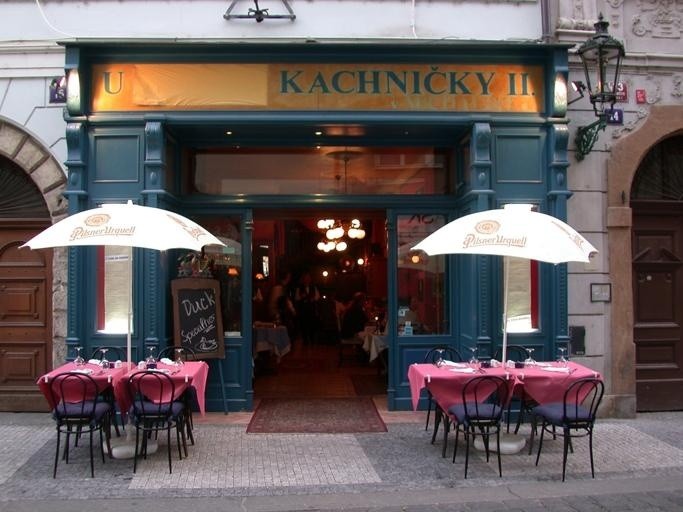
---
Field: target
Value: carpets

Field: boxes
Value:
[245,397,388,433]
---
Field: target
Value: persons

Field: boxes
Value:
[292,271,321,348]
[271,265,297,349]
[338,291,371,363]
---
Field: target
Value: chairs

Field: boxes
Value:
[50,346,198,474]
[424,344,604,483]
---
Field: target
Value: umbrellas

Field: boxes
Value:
[394,236,446,334]
[409,204,599,367]
[16,198,229,370]
[200,234,243,268]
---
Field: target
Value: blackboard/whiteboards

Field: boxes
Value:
[171,278,225,360]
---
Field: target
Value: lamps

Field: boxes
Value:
[568,10,626,161]
[53,76,65,99]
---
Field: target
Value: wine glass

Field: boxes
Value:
[524,348,537,369]
[175,348,185,369]
[144,346,157,369]
[436,349,447,369]
[469,347,480,369]
[558,347,568,368]
[73,347,86,368]
[98,349,111,370]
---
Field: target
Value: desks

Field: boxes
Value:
[410,361,513,459]
